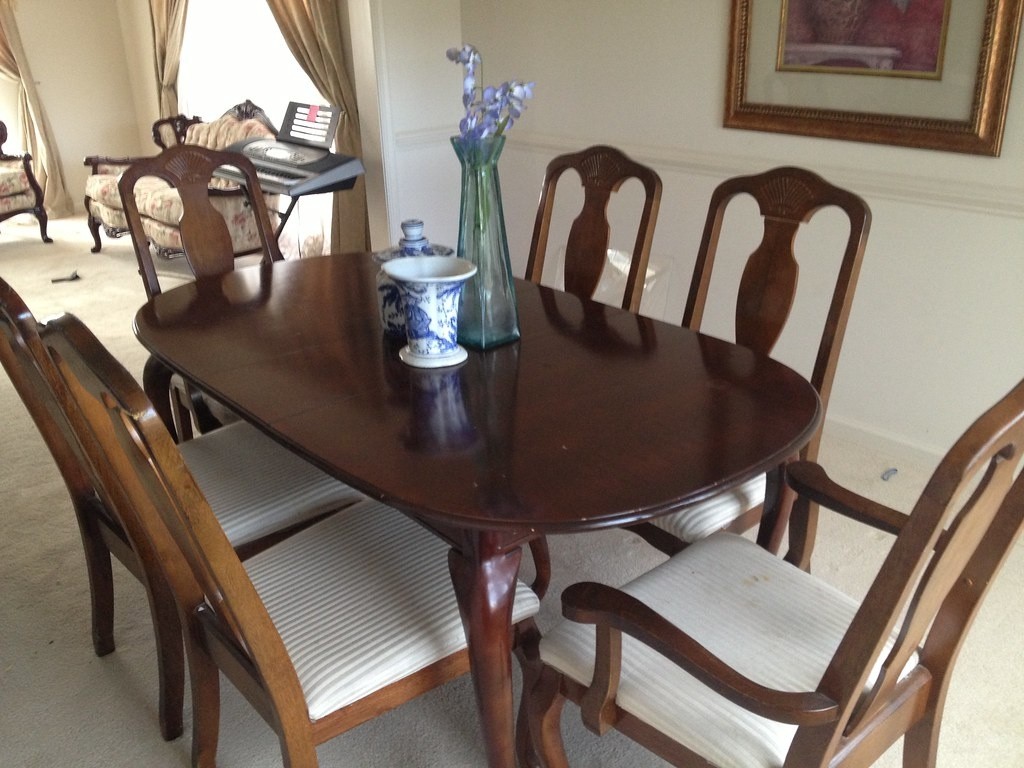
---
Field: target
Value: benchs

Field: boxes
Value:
[82,98,278,260]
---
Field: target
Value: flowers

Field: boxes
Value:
[445,42,535,140]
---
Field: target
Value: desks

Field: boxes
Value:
[132,253,823,768]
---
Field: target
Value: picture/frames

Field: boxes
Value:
[721,0,1024,158]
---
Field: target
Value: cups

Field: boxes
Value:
[383,256,477,368]
[371,220,454,337]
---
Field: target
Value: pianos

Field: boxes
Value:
[210,99,366,241]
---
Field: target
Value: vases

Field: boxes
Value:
[448,133,522,351]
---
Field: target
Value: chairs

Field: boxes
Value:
[39,310,542,768]
[527,378,1024,768]
[525,143,664,315]
[0,120,55,244]
[116,144,286,443]
[524,167,874,574]
[0,279,367,742]
